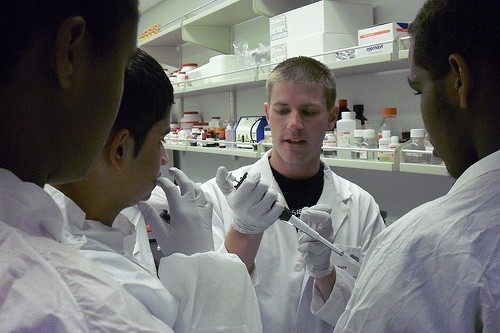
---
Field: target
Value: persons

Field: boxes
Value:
[323,0,500,333]
[198,55,386,333]
[39,47,264,333]
[0,0,179,333]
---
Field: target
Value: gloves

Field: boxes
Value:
[297,203,333,277]
[216,166,284,234]
[138,166,215,256]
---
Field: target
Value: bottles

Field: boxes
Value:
[176,68,186,88]
[336,111,361,159]
[180,111,202,130]
[376,108,402,143]
[322,128,337,158]
[169,68,178,90]
[225,123,234,148]
[352,105,368,130]
[206,130,226,148]
[351,130,365,160]
[403,128,426,163]
[360,129,378,160]
[379,130,399,162]
[334,99,352,128]
[209,116,222,127]
[181,63,199,74]
[167,122,180,144]
[163,69,170,79]
[177,122,208,146]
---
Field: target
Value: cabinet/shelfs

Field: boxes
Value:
[134,0,451,180]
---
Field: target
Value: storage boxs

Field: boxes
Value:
[357,22,411,56]
[270,33,358,73]
[268,0,376,44]
